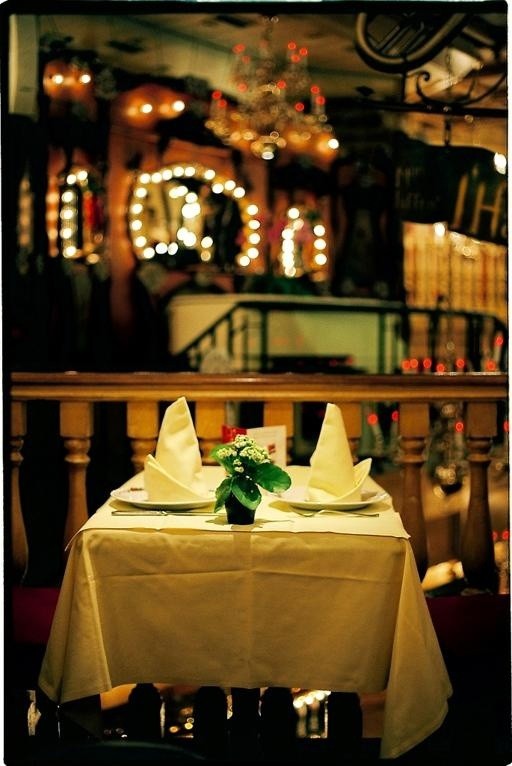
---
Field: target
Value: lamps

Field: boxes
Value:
[206,12,341,165]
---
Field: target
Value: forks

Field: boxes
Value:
[291,506,379,517]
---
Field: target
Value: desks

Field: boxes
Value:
[35,466,453,766]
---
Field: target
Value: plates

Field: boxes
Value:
[112,486,217,511]
[268,491,385,508]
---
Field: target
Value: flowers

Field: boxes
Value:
[210,434,291,511]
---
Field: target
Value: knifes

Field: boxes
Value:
[111,511,220,517]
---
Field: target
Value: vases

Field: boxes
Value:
[224,492,254,525]
